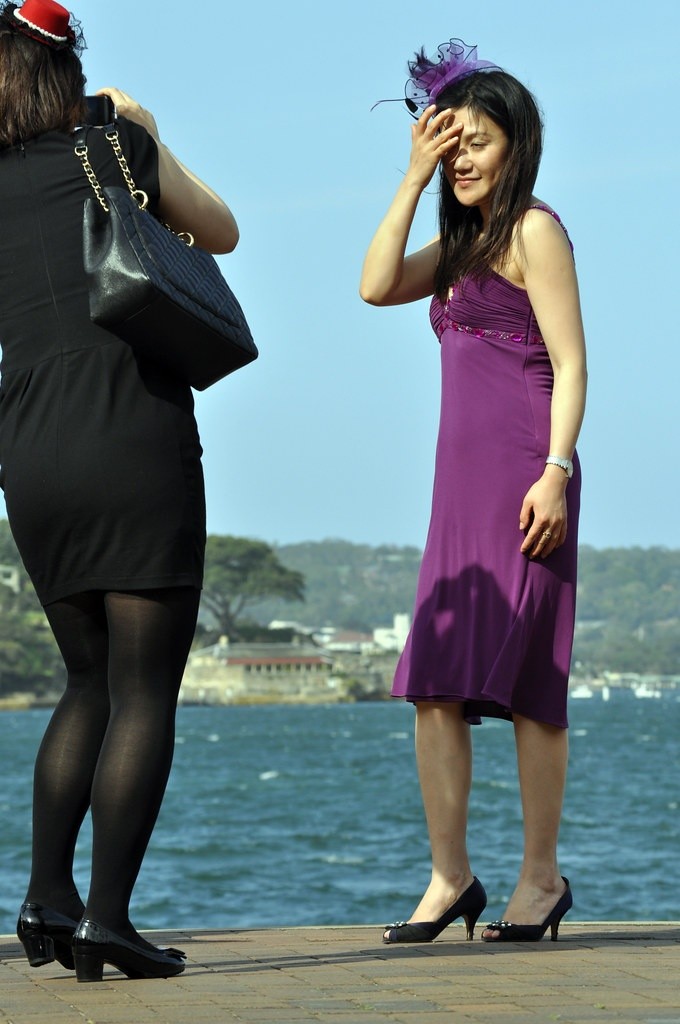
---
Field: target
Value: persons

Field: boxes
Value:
[0,0,239,977]
[361,37,586,943]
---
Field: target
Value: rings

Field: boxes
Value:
[542,532,551,539]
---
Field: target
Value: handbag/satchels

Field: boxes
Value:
[74,123,259,390]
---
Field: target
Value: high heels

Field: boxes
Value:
[71,920,188,983]
[480,876,573,943]
[15,903,85,969]
[382,876,487,943]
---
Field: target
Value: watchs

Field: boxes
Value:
[545,456,574,478]
